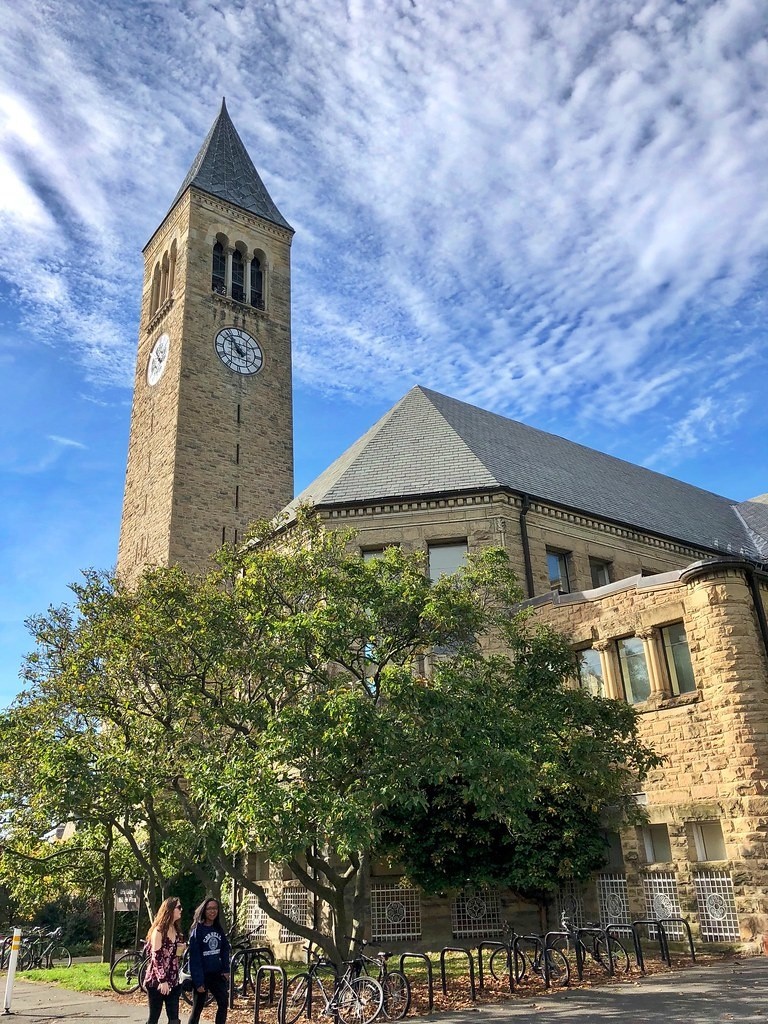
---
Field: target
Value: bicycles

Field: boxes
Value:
[342,934,411,1022]
[0,924,72,972]
[549,909,630,976]
[110,919,275,1006]
[278,946,383,1024]
[489,919,570,988]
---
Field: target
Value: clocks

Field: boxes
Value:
[145,332,170,388]
[214,326,263,375]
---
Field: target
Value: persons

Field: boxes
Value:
[144,897,186,1024]
[188,897,231,1024]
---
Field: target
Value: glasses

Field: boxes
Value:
[206,907,218,911]
[174,905,182,910]
